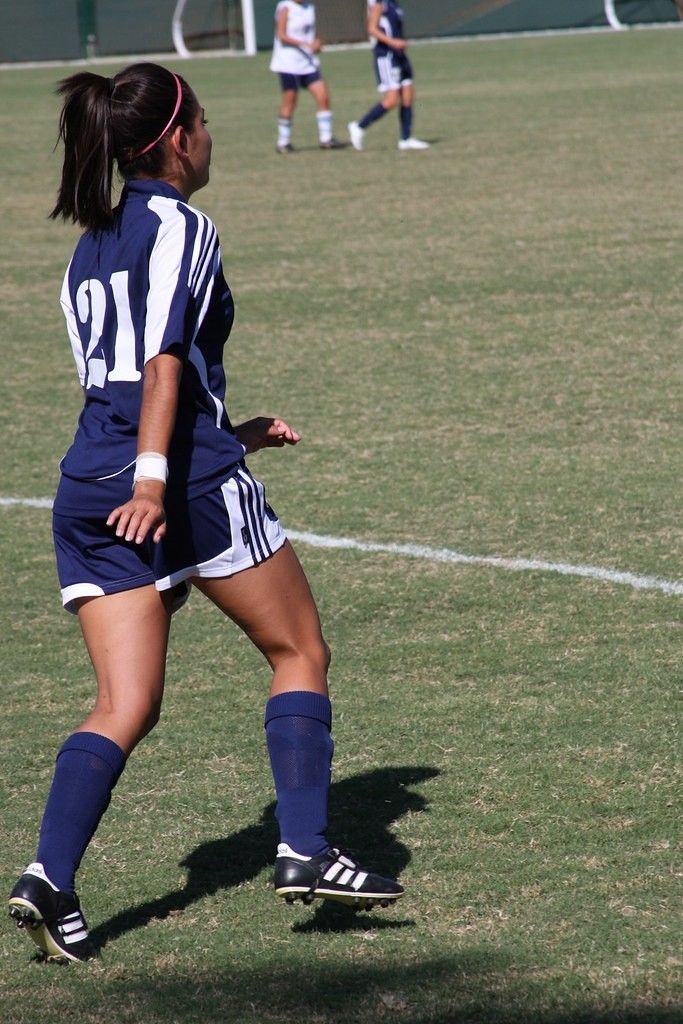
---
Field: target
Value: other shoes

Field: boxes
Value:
[319,138,348,149]
[275,144,295,154]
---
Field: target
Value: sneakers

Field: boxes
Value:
[398,137,430,151]
[347,121,365,151]
[273,842,405,911]
[8,863,101,963]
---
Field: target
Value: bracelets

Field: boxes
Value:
[132,451,169,485]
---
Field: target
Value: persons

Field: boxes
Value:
[269,1,348,155]
[5,62,406,964]
[347,0,431,152]
